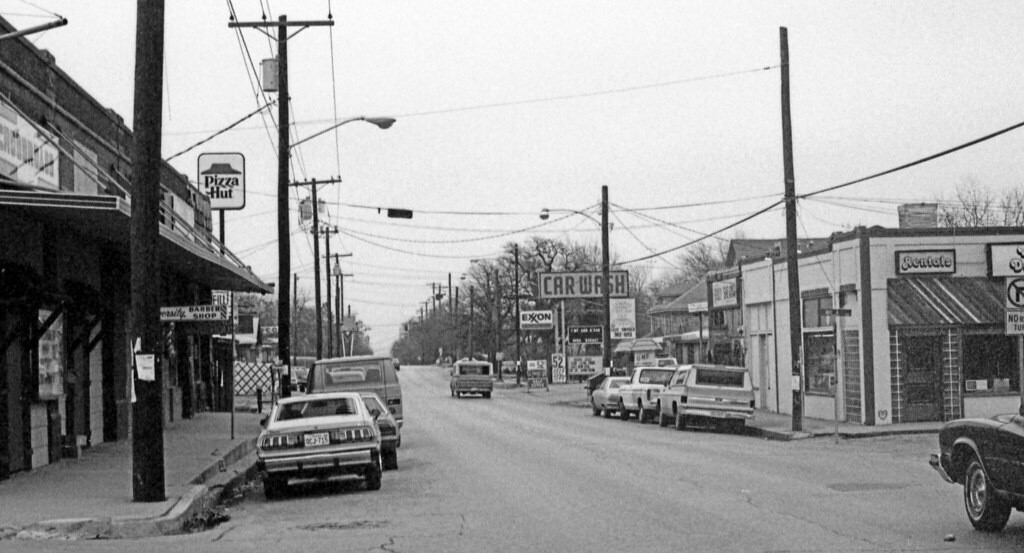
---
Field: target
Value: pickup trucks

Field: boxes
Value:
[618,366,678,422]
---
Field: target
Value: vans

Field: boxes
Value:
[656,360,755,433]
[306,355,404,448]
[450,363,494,399]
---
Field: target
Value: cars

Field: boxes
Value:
[257,392,384,499]
[814,373,835,392]
[301,392,401,470]
[392,359,400,370]
[928,408,1024,532]
[283,365,368,392]
[589,375,630,417]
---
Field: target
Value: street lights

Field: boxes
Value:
[277,114,395,398]
[540,206,611,375]
[470,258,521,386]
